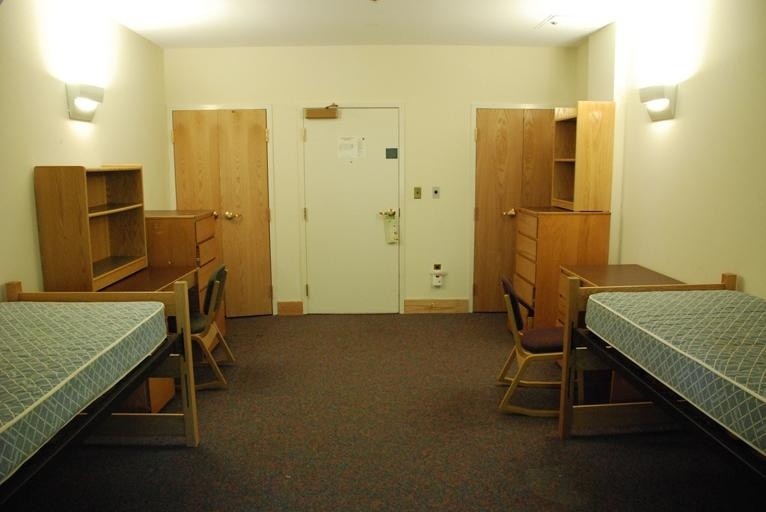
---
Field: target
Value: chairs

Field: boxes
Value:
[498,275,590,417]
[167,265,236,391]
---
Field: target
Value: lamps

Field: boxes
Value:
[67,85,103,122]
[640,86,677,123]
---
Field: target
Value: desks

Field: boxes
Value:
[557,264,689,403]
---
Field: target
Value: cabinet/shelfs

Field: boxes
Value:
[551,100,615,212]
[505,206,610,335]
[82,210,226,413]
[34,164,148,293]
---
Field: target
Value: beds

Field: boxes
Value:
[0,282,198,512]
[558,272,766,476]
